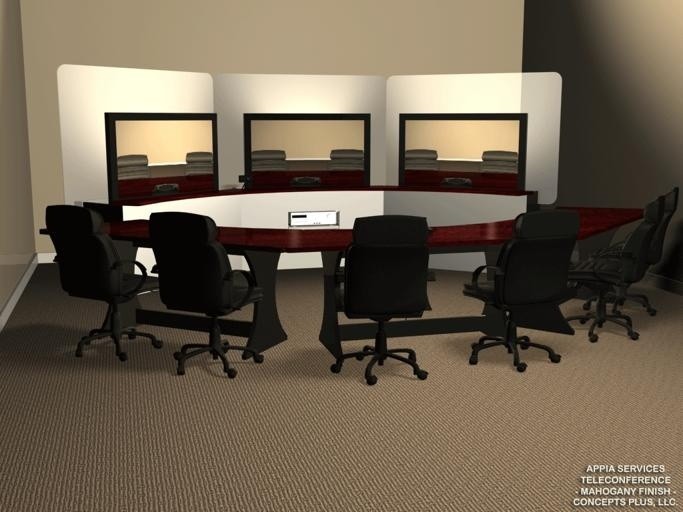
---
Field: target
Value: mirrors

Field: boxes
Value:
[104,109,219,207]
[396,111,529,187]
[241,111,371,189]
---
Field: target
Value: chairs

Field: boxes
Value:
[461,185,680,372]
[331,215,437,386]
[44,204,263,378]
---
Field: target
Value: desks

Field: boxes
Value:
[39,206,645,361]
